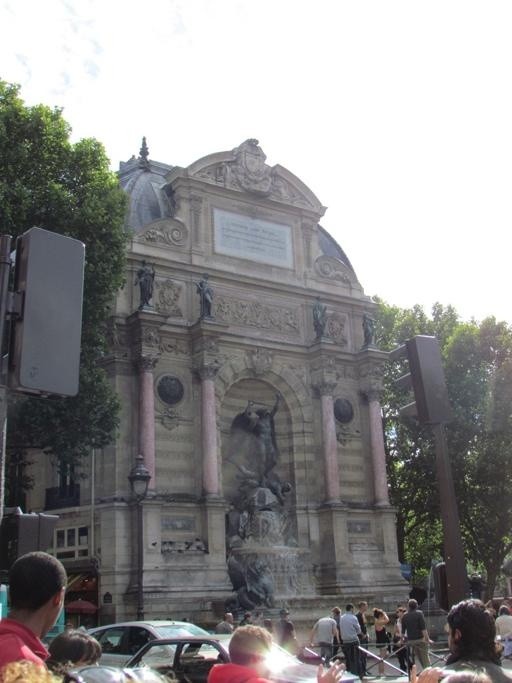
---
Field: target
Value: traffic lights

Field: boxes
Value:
[388,341,419,422]
[3,512,61,582]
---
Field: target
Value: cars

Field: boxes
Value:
[62,633,360,682]
[85,620,212,666]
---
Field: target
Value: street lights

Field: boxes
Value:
[427,557,439,638]
[125,452,153,619]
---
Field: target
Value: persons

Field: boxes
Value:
[243,390,282,485]
[361,311,377,345]
[209,624,346,682]
[485,599,511,658]
[216,612,233,633]
[438,598,511,682]
[307,598,433,683]
[311,300,328,339]
[48,630,102,672]
[1,552,67,681]
[195,277,215,317]
[223,455,293,502]
[239,610,296,646]
[132,259,158,306]
